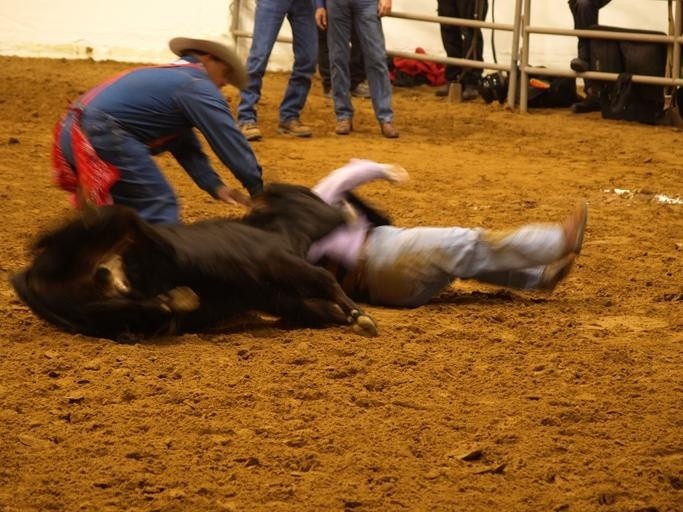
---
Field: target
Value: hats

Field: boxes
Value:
[170,31,248,91]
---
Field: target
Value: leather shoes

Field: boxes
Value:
[571,96,599,114]
[435,83,450,97]
[570,58,589,71]
[461,85,478,100]
[540,258,573,291]
[563,200,588,254]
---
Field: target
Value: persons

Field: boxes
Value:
[566,0,612,114]
[311,0,400,139]
[315,24,373,99]
[304,156,590,309]
[50,28,265,221]
[236,0,318,141]
[435,1,488,100]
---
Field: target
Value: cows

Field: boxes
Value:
[9,177,391,347]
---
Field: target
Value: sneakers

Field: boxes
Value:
[278,120,311,136]
[239,122,261,140]
[335,119,351,135]
[379,122,398,137]
